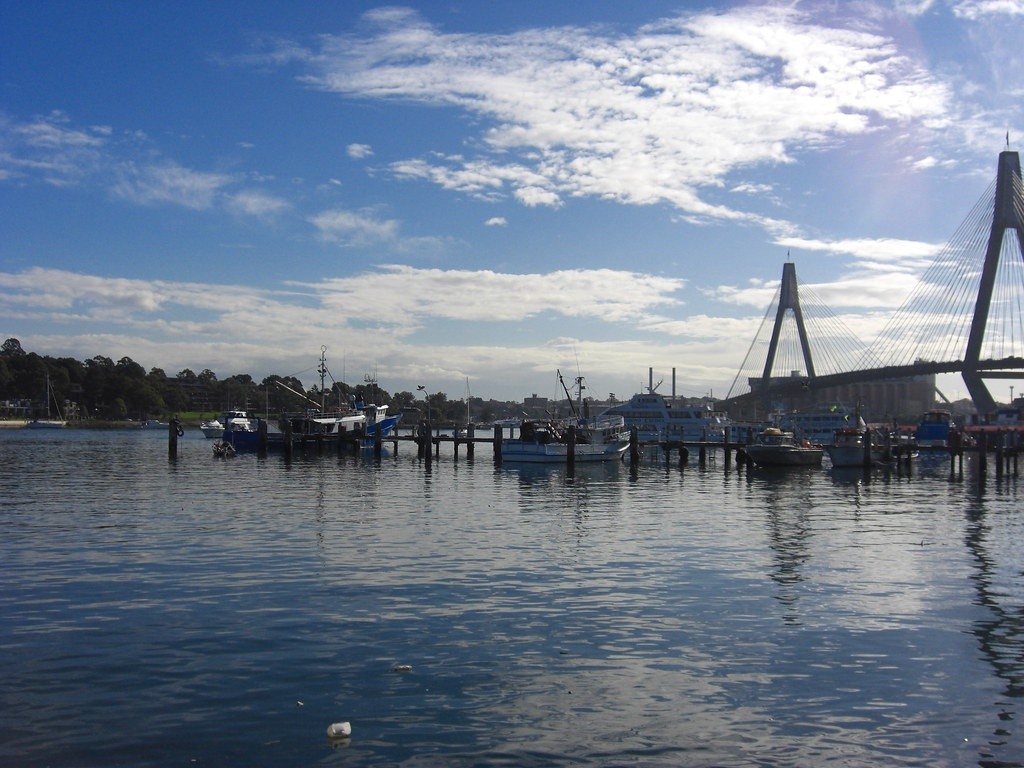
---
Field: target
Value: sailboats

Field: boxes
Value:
[27,375,72,428]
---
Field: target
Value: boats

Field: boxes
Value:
[142,419,170,429]
[887,428,921,461]
[200,402,257,439]
[500,369,632,462]
[915,410,977,451]
[223,345,406,449]
[595,380,734,443]
[730,402,888,468]
